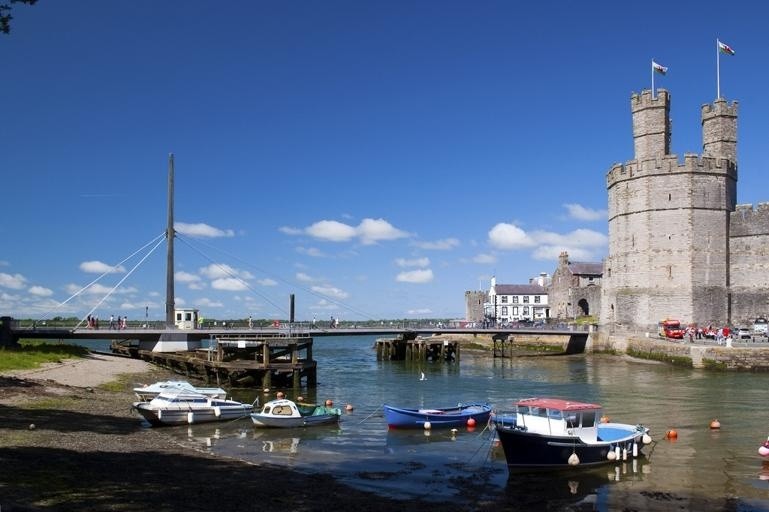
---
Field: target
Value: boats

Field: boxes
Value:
[382,397,650,468]
[132,380,342,428]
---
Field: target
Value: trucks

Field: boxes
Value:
[658,319,684,339]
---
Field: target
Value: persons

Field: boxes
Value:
[84,312,130,331]
[198,316,205,328]
[245,315,255,329]
[684,322,730,345]
[329,316,337,329]
[311,316,319,328]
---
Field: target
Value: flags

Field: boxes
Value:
[719,41,739,57]
[650,61,669,76]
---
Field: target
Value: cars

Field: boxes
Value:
[733,327,751,339]
